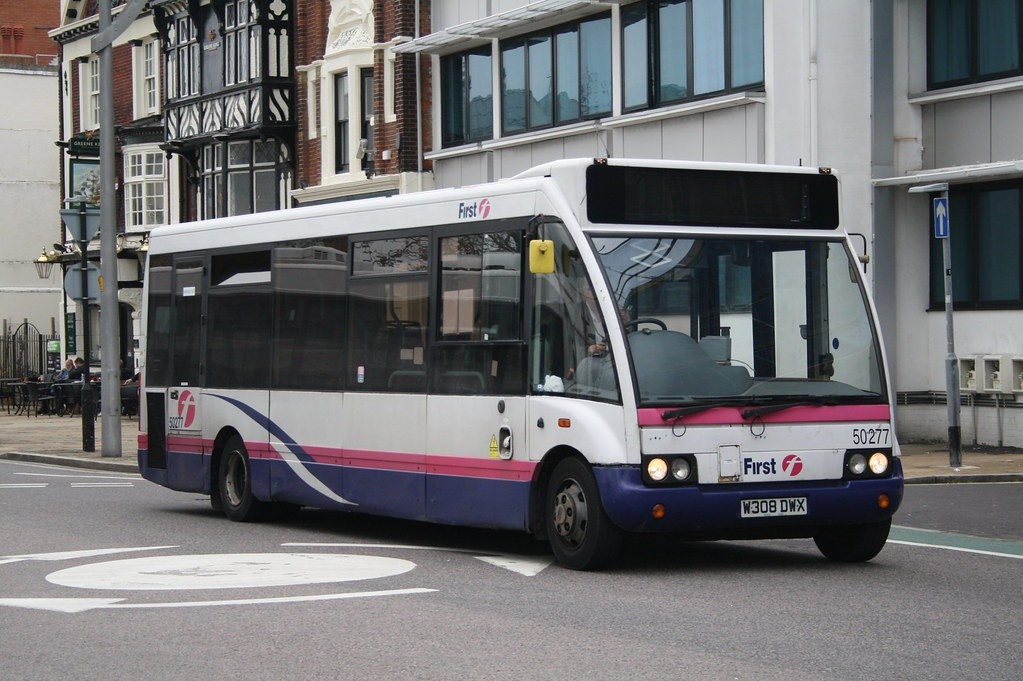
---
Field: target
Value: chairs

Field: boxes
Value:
[0,379,99,420]
[373,319,502,394]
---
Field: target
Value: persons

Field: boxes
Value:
[124,371,141,417]
[53,357,90,416]
[542,255,637,383]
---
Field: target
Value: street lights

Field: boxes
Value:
[908,181,962,467]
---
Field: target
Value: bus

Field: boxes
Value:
[136,156,906,571]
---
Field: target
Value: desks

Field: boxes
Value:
[0,377,139,417]
